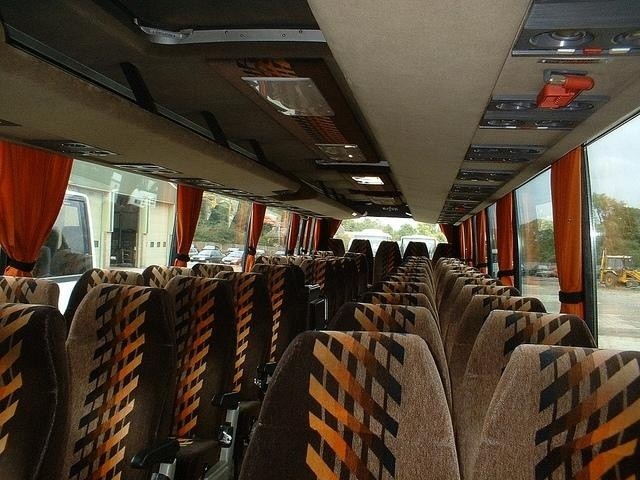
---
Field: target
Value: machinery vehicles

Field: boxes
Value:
[598,253,640,289]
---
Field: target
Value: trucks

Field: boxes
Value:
[2,157,174,314]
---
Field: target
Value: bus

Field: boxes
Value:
[348,228,391,258]
[400,235,438,261]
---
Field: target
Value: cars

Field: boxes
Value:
[514,260,600,280]
[190,244,287,257]
[221,252,245,266]
[190,250,223,264]
